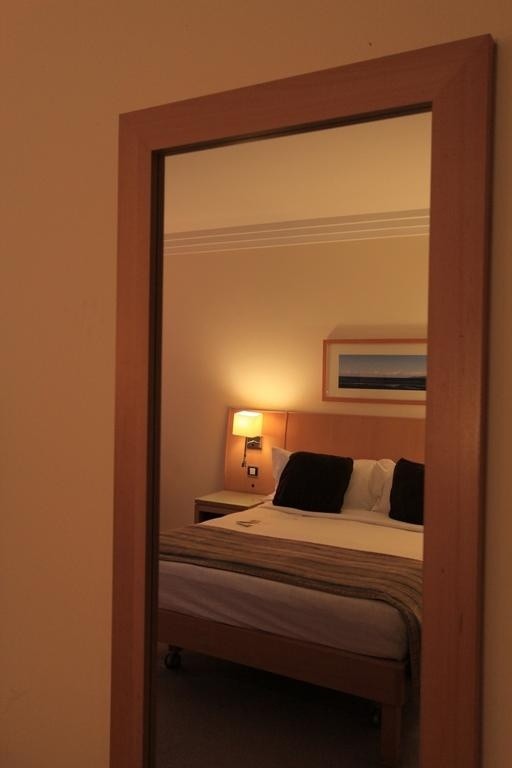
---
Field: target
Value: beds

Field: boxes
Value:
[157,411,427,765]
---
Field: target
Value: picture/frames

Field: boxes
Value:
[321,337,427,405]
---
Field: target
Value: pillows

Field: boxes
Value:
[271,447,424,526]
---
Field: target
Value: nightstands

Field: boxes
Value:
[194,491,265,524]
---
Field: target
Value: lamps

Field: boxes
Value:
[232,410,262,467]
